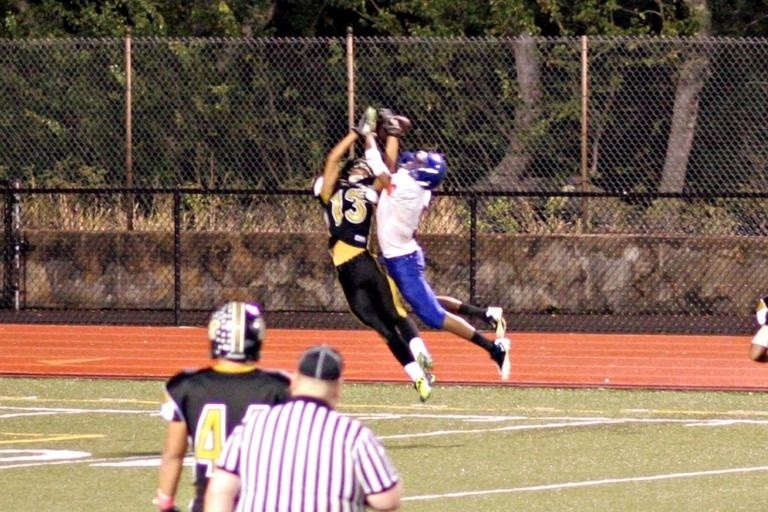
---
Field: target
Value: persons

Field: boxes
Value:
[155,301,295,511]
[365,107,511,384]
[750,296,767,362]
[314,109,435,401]
[204,345,402,512]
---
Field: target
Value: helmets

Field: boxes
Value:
[208,302,264,361]
[402,151,445,189]
[341,159,373,177]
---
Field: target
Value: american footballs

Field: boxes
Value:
[379,116,410,138]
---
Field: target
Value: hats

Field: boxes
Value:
[299,344,342,380]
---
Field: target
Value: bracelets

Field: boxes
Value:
[155,488,175,509]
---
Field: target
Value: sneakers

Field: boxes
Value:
[412,306,510,401]
